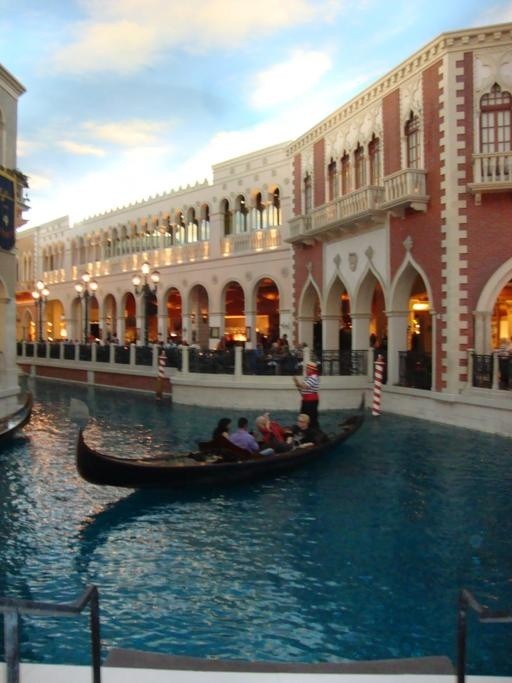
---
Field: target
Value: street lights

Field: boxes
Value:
[32,281,49,342]
[131,262,160,346]
[73,273,98,344]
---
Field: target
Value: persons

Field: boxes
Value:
[86,330,202,353]
[213,412,330,459]
[217,332,292,374]
[291,360,330,442]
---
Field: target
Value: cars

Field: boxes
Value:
[210,347,321,375]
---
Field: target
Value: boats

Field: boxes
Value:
[76,391,365,489]
[0,387,34,434]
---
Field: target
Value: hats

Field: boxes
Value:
[306,362,318,371]
